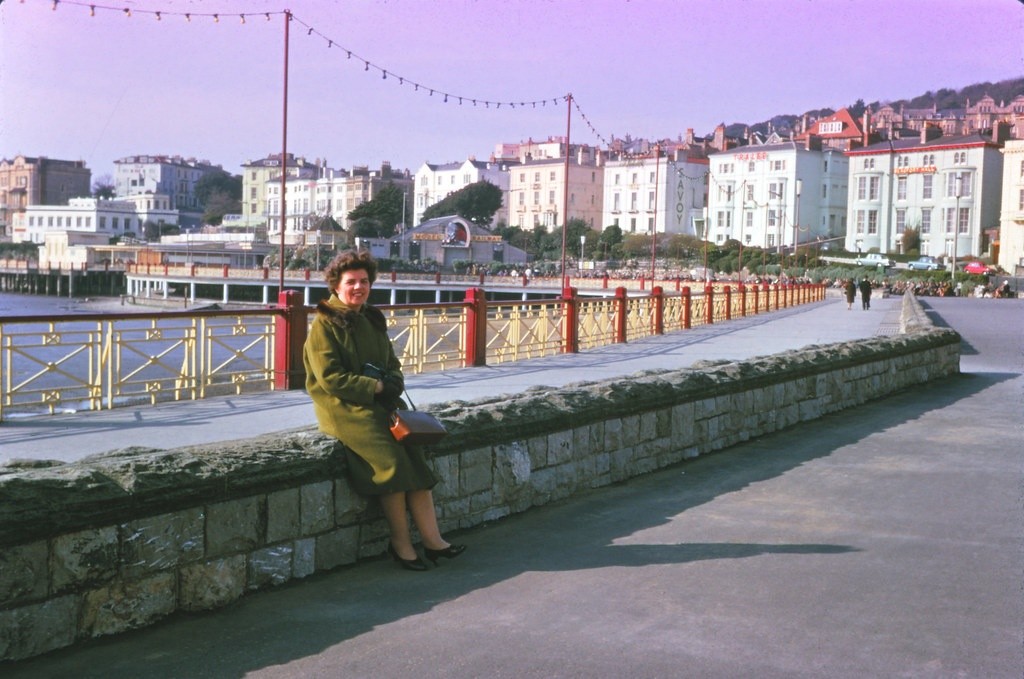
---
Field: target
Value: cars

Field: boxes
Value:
[857,253,897,268]
[965,259,996,276]
[908,255,947,271]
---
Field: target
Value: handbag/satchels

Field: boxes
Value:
[388,378,447,445]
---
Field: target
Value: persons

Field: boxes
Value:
[822,278,843,288]
[544,269,556,277]
[845,277,856,310]
[707,266,813,286]
[860,276,872,310]
[909,281,949,296]
[465,264,532,286]
[654,266,706,282]
[869,278,907,296]
[576,269,603,279]
[302,251,467,573]
[981,280,1010,299]
[608,269,652,280]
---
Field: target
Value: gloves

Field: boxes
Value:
[381,379,403,397]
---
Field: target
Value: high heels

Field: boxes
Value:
[425,544,467,566]
[387,540,427,571]
